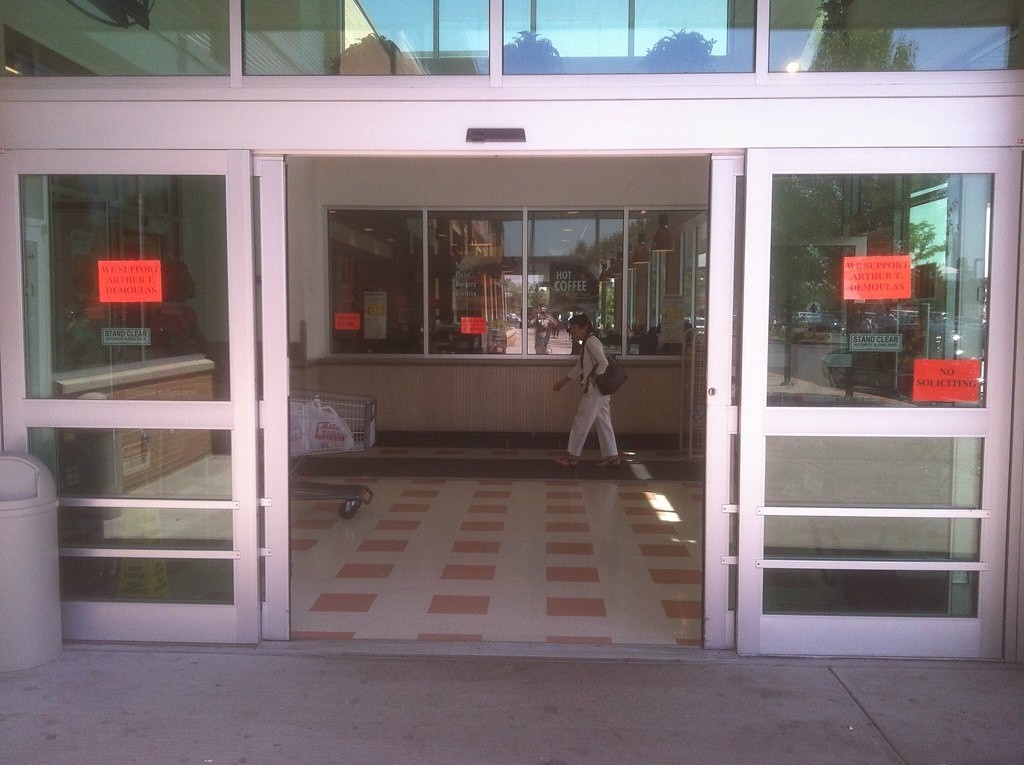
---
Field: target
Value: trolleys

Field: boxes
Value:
[288,387,377,518]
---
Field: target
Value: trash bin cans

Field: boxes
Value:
[75,391,123,521]
[1,448,62,676]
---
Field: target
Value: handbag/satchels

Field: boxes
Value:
[586,336,629,396]
[289,396,354,453]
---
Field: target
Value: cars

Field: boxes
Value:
[790,310,988,398]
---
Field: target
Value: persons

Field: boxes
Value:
[553,313,620,467]
[529,306,558,354]
